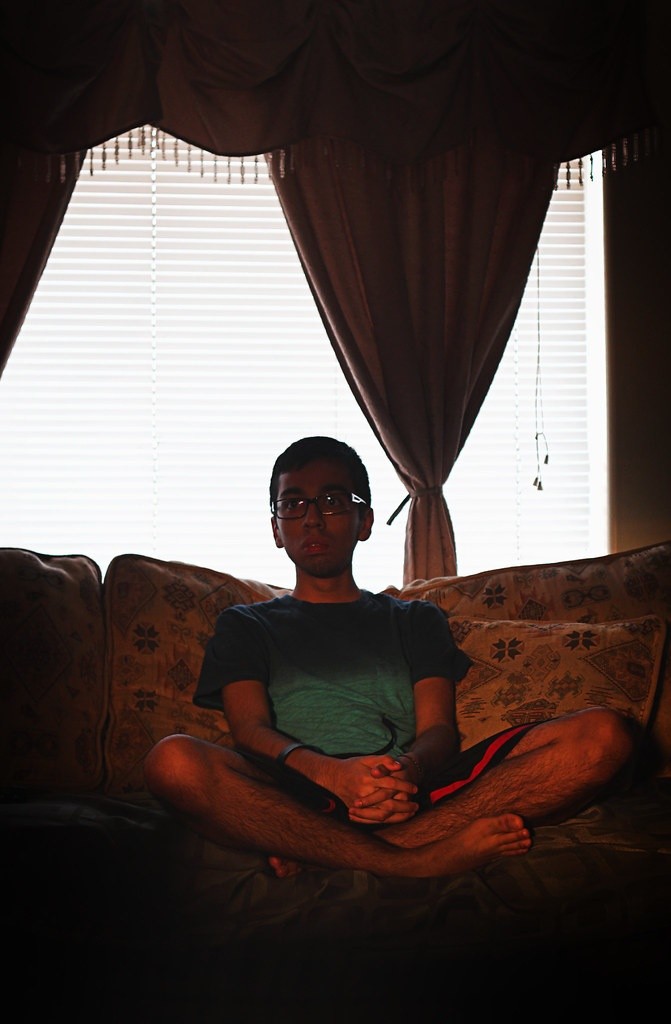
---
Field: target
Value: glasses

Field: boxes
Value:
[269,492,369,521]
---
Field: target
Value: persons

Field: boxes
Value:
[142,437,630,880]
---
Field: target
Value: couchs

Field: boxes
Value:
[0,778,670,1022]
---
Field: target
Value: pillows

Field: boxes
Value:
[103,553,298,818]
[0,548,107,796]
[398,539,670,781]
[441,613,666,797]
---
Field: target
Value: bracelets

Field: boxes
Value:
[272,740,322,776]
